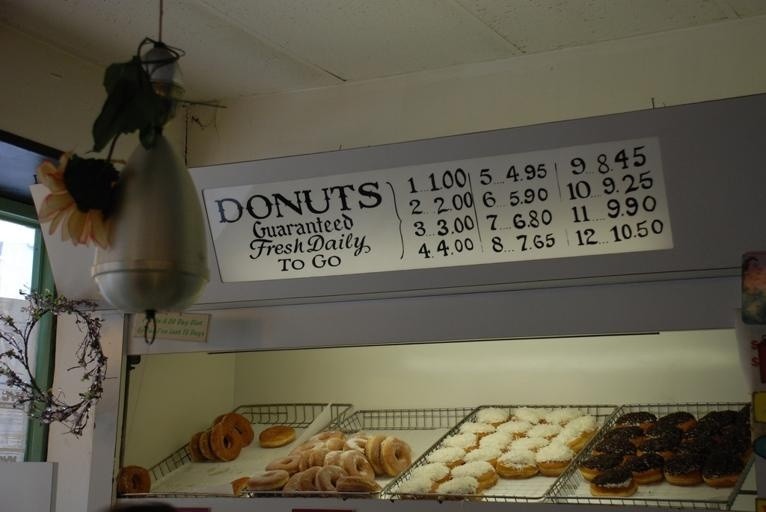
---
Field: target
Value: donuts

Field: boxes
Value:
[579,411,754,497]
[190,413,412,492]
[117,466,151,497]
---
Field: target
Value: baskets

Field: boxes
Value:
[383,404,618,504]
[239,406,476,500]
[119,402,753,510]
[120,403,352,499]
[545,403,754,512]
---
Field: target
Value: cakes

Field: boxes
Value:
[389,407,597,501]
